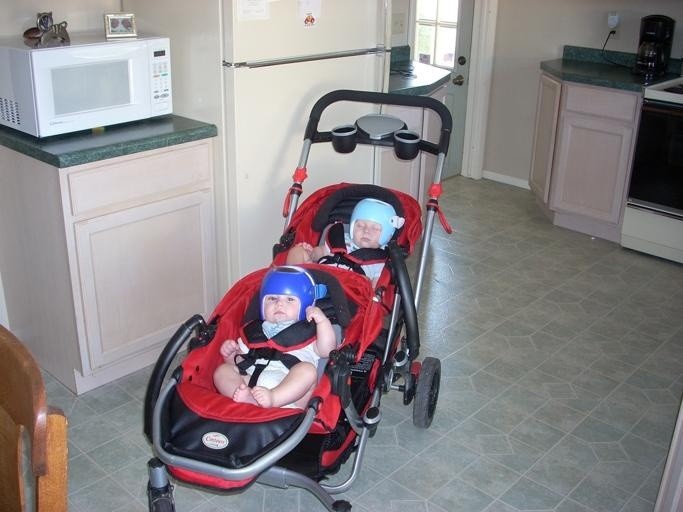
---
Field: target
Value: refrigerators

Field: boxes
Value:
[136,1,393,303]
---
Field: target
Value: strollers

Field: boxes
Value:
[141,88,452,512]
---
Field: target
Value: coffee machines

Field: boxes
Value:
[634,14,676,80]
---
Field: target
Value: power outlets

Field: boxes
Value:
[601,14,621,39]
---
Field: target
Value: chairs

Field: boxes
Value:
[0,325,68,512]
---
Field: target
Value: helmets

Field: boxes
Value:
[349,198,398,247]
[259,265,318,322]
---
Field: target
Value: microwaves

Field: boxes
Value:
[1,26,175,140]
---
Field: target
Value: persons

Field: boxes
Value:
[211,266,336,410]
[286,196,398,308]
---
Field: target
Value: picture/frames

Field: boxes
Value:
[104,12,138,38]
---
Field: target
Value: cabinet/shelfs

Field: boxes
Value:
[529,72,643,244]
[381,84,449,238]
[0,138,217,397]
